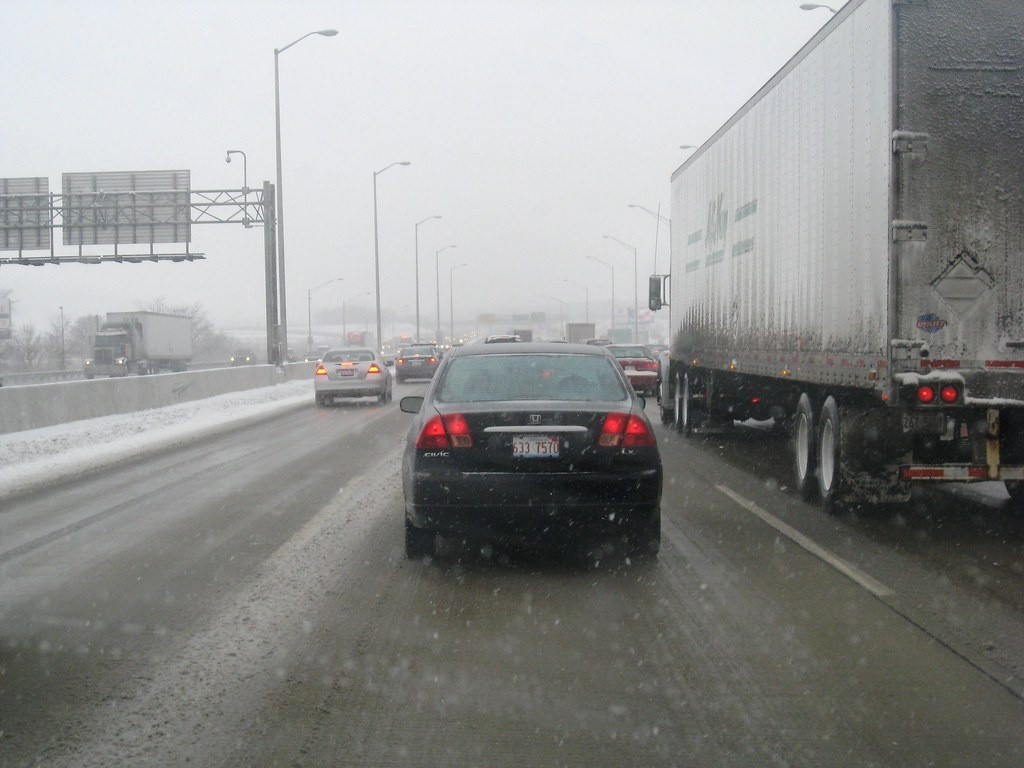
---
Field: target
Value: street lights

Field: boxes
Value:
[373,160,411,356]
[226,148,249,221]
[415,215,443,342]
[59,305,65,370]
[308,276,344,361]
[272,27,341,363]
[603,234,639,345]
[435,245,456,337]
[450,262,468,339]
[584,254,617,342]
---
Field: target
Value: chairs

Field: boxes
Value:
[556,375,590,394]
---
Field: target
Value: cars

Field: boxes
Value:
[312,345,394,408]
[398,340,667,562]
[229,349,256,367]
[286,321,670,396]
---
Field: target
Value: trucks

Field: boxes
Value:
[81,311,193,380]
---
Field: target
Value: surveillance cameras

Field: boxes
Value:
[226,157,231,163]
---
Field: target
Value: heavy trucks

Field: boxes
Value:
[645,0,1024,519]
